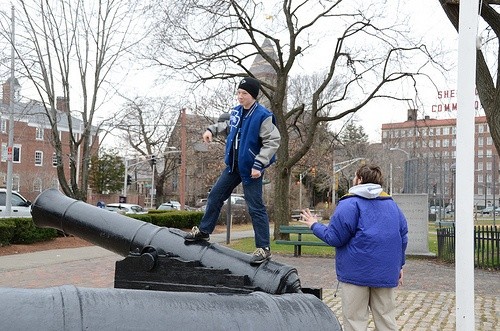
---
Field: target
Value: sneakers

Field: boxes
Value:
[249,247,272,263]
[184,226,210,241]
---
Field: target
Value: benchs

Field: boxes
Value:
[275,225,331,257]
[290,208,325,221]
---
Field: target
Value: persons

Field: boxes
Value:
[298,163,408,331]
[184,77,282,265]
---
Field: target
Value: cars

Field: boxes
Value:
[105,203,148,215]
[195,195,245,213]
[0,187,33,219]
[475,202,500,216]
[156,201,191,212]
[429,205,455,215]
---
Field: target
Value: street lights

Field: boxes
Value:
[150,146,178,209]
[150,155,157,209]
[332,150,370,205]
[388,147,410,193]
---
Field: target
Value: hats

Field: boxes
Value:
[237,78,260,99]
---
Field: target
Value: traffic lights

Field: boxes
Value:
[296,179,299,185]
[433,184,437,193]
